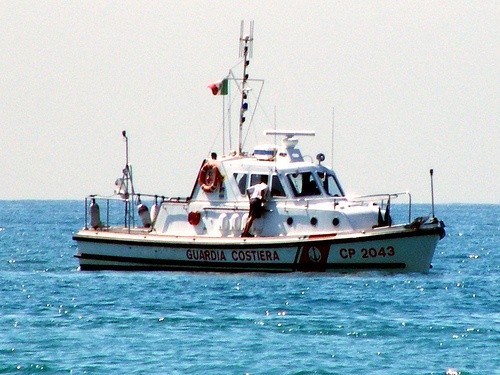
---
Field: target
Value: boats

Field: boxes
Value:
[73,17,447,274]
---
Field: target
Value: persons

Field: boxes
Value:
[240,178,268,238]
[210,151,217,160]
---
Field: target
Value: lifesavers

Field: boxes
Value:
[198,163,220,192]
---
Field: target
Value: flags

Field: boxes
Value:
[209,78,229,96]
